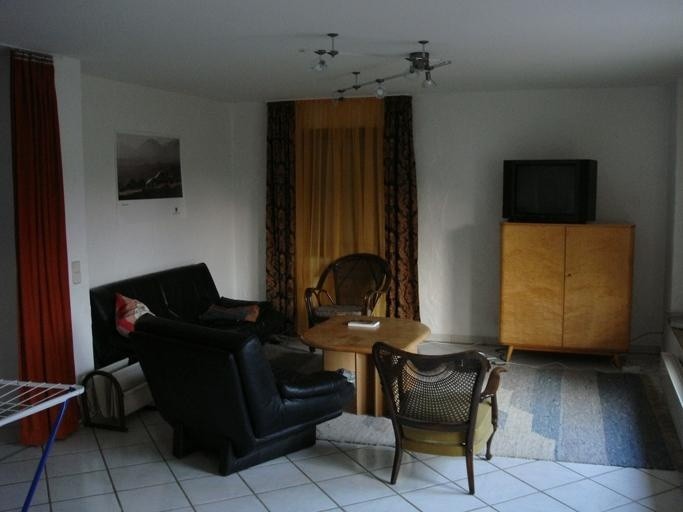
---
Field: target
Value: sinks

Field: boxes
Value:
[89,263,290,408]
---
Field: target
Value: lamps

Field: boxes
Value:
[372,342,508,493]
[303,253,392,328]
[128,313,355,476]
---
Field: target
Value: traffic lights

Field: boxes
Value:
[114,291,156,337]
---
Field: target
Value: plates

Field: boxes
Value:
[500,221,635,368]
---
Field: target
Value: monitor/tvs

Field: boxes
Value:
[348,320,380,327]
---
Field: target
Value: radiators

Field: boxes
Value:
[347,319,380,327]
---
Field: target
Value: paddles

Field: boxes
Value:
[272,350,683,469]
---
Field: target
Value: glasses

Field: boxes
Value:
[299,33,451,103]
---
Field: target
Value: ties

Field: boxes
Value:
[502,158,598,224]
